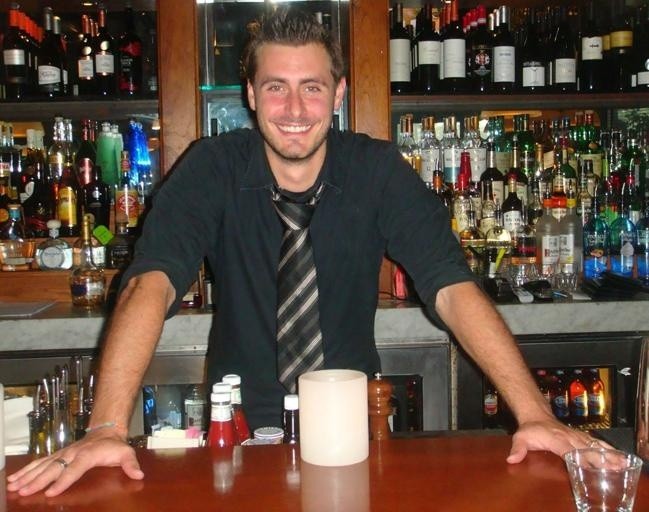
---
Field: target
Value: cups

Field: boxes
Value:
[297,370,372,467]
[299,457,371,512]
[563,449,644,512]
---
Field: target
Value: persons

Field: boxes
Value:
[5,7,628,498]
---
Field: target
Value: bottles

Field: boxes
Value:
[366,373,420,442]
[481,366,607,427]
[382,0,649,92]
[283,450,299,492]
[141,387,159,435]
[389,105,645,302]
[1,119,155,270]
[69,216,109,309]
[24,356,98,457]
[2,0,158,101]
[177,267,213,312]
[183,372,298,449]
[208,452,244,495]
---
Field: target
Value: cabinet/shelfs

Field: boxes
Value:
[0,0,649,440]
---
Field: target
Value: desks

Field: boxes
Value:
[0,440,649,512]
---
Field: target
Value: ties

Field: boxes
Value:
[266,185,329,394]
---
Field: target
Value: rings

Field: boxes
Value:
[588,440,599,448]
[54,457,69,468]
